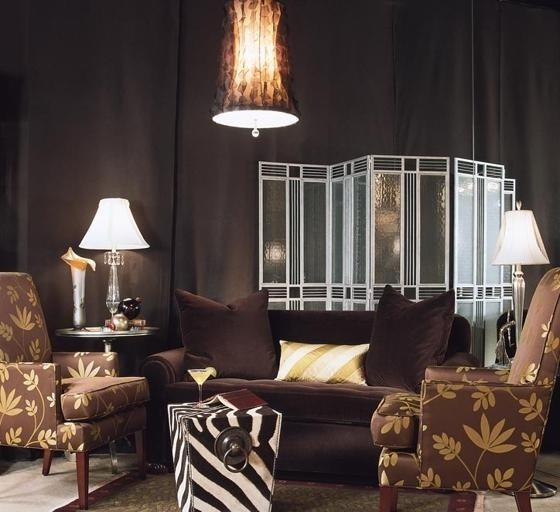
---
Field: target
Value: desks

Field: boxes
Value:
[55,327,161,352]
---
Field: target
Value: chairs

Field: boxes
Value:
[496,309,560,454]
[0,272,151,509]
[370,267,560,512]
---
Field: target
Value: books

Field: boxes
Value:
[203,387,268,412]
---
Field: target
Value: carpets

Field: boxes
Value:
[53,471,477,512]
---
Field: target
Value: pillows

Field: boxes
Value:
[364,285,455,394]
[175,289,278,382]
[274,339,370,387]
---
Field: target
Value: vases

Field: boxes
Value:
[61,247,96,332]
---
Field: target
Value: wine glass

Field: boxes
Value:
[187,369,215,409]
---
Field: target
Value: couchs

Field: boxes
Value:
[140,310,482,485]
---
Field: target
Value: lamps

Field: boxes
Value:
[208,1,301,137]
[78,198,150,318]
[490,201,557,499]
